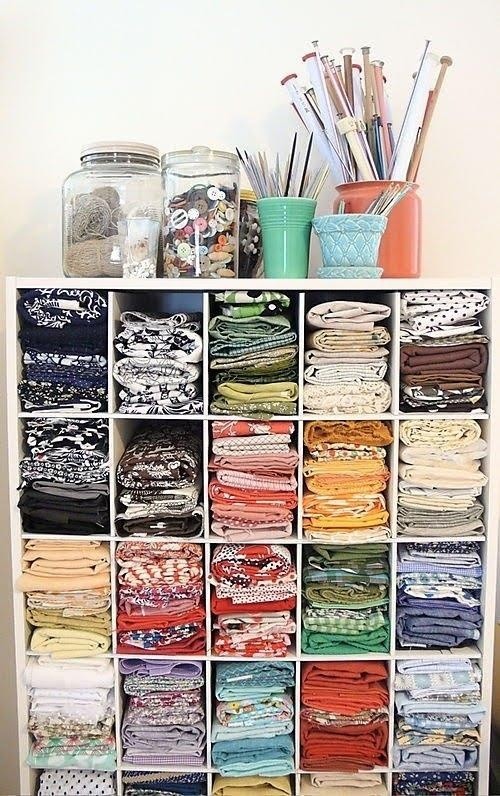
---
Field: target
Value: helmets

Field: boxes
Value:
[315,262,386,281]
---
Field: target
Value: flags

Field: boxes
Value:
[311,214,391,279]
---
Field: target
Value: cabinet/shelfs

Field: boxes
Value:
[60,138,165,279]
[153,146,242,278]
[237,184,267,279]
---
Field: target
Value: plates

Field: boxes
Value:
[4,273,500,796]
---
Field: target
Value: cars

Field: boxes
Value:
[334,179,423,280]
[255,194,318,281]
[304,213,390,268]
